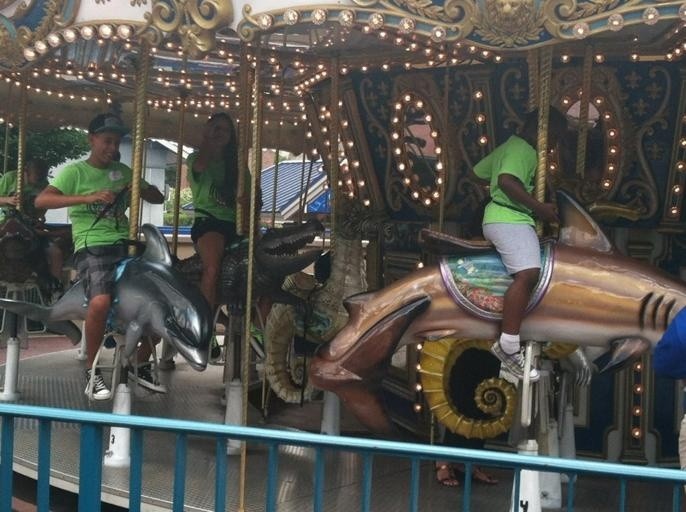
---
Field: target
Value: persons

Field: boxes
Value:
[1,158,63,281]
[186,113,274,360]
[470,105,567,382]
[432,348,499,487]
[34,113,168,400]
[653,305,686,497]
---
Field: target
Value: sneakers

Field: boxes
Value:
[127,363,169,394]
[490,338,541,383]
[81,367,112,400]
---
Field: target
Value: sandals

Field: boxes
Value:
[453,462,501,485]
[432,459,459,488]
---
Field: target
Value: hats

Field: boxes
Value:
[88,113,132,137]
